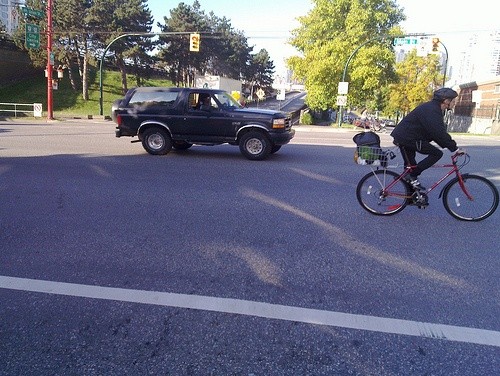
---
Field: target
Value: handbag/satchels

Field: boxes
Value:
[352,131,381,165]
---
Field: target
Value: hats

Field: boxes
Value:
[434,88,459,101]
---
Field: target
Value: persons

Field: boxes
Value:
[390,87,464,206]
[197,94,215,111]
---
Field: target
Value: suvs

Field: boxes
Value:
[111,87,296,159]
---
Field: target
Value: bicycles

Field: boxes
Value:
[355,142,499,222]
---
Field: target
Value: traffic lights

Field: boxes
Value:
[189,33,201,52]
[432,37,439,51]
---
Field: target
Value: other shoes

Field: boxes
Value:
[404,179,426,191]
[406,192,427,201]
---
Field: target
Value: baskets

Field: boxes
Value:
[355,146,389,169]
